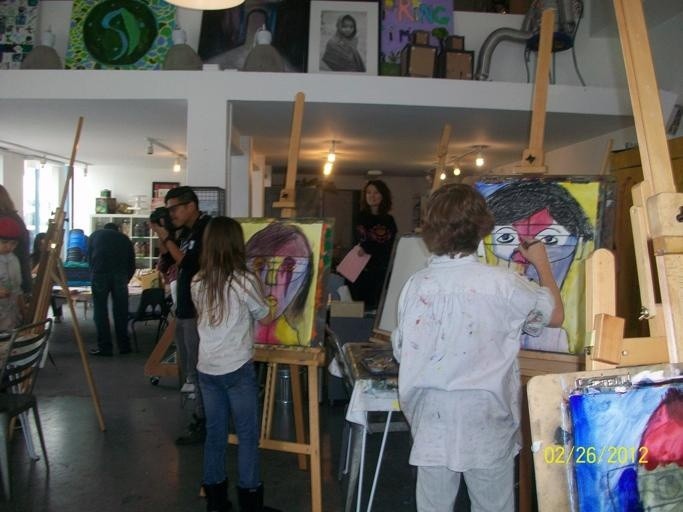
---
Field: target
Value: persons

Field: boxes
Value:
[0,218,22,388]
[396,183,565,512]
[320,13,366,72]
[136,247,179,321]
[189,217,274,512]
[88,223,137,357]
[350,178,396,314]
[31,232,63,324]
[151,186,214,446]
[0,181,33,326]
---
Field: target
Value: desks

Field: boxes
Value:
[52,286,170,342]
[328,341,400,512]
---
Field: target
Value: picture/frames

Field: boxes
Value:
[308,0,379,75]
[152,182,180,211]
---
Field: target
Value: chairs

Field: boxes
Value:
[129,288,166,347]
[323,323,411,481]
[521,0,585,86]
[0,318,52,500]
[66,229,88,261]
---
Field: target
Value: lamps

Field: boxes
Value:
[327,141,336,162]
[145,141,155,155]
[453,161,462,176]
[173,157,181,172]
[475,146,485,167]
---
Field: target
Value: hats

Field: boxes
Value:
[0,217,22,240]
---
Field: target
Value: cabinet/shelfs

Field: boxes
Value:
[609,136,683,336]
[90,215,160,270]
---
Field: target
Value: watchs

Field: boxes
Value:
[161,233,173,247]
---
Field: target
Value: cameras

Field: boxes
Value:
[150,207,174,233]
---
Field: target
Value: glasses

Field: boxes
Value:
[165,203,187,211]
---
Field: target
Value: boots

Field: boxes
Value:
[202,478,234,512]
[237,482,283,512]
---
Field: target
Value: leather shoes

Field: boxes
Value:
[174,418,206,445]
[88,348,102,356]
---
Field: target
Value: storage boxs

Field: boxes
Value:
[63,262,92,286]
[96,198,116,214]
